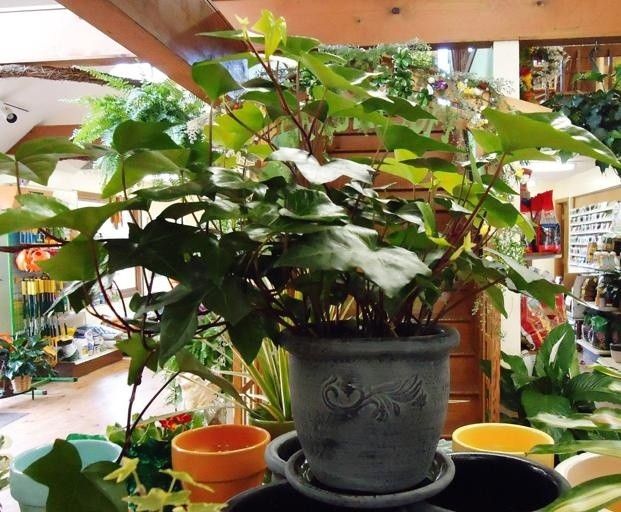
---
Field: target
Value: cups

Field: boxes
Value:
[453,421,556,469]
[556,440,621,511]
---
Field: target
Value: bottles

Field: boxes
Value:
[600,297,606,307]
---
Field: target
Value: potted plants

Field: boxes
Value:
[1,329,55,392]
[581,312,593,340]
[0,14,621,492]
[157,271,361,441]
[589,313,609,349]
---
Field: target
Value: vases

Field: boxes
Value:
[259,425,297,481]
[441,420,558,474]
[209,482,453,511]
[557,449,620,512]
[432,450,570,512]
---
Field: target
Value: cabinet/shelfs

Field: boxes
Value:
[566,268,620,368]
[565,202,621,275]
